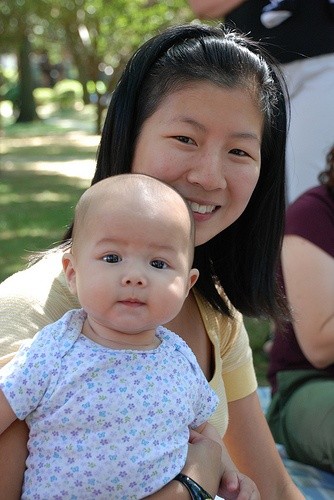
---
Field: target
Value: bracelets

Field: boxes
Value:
[173,474,214,500]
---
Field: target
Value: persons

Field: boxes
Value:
[0,25,307,500]
[0,172,219,500]
[264,147,334,472]
[186,1,333,400]
